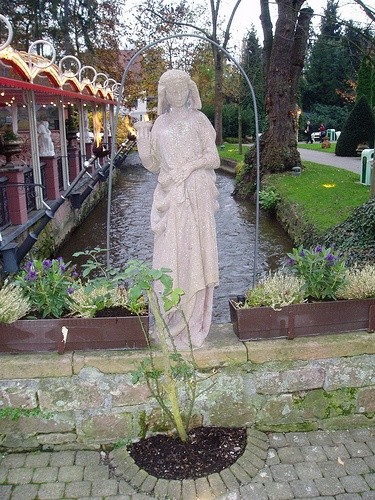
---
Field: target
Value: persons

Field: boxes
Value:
[318,123,327,143]
[132,68,221,352]
[318,125,327,131]
[304,120,314,144]
